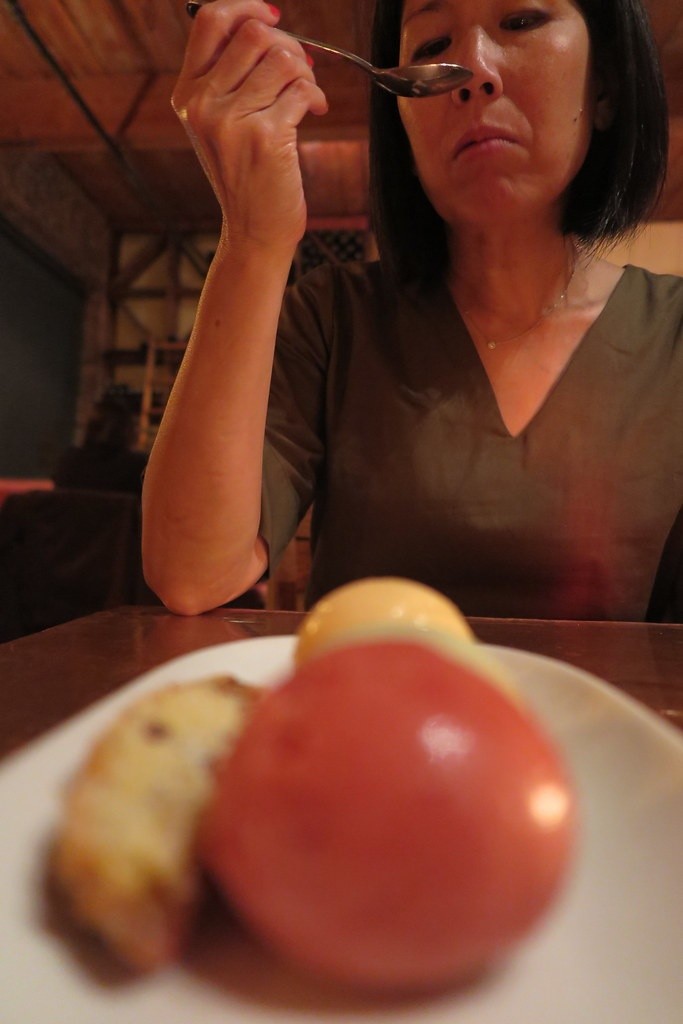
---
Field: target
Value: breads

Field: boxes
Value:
[48,670,259,975]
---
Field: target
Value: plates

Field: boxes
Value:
[1,634,682,1024]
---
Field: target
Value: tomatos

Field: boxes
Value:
[207,641,575,990]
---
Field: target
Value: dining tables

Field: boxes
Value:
[0,605,683,1024]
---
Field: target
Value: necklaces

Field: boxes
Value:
[453,236,579,350]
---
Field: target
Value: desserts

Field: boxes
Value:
[292,575,489,674]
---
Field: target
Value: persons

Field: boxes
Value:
[134,0,682,621]
[51,382,147,498]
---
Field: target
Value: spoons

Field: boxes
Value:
[185,1,474,98]
